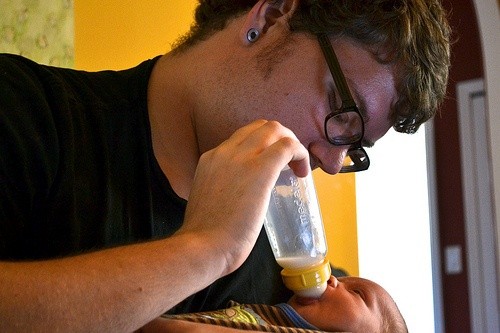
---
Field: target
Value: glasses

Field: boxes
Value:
[314,32,370,173]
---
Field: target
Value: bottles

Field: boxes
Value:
[262,163,332,299]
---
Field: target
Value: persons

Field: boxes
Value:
[132,276,408,333]
[0,0,452,333]
